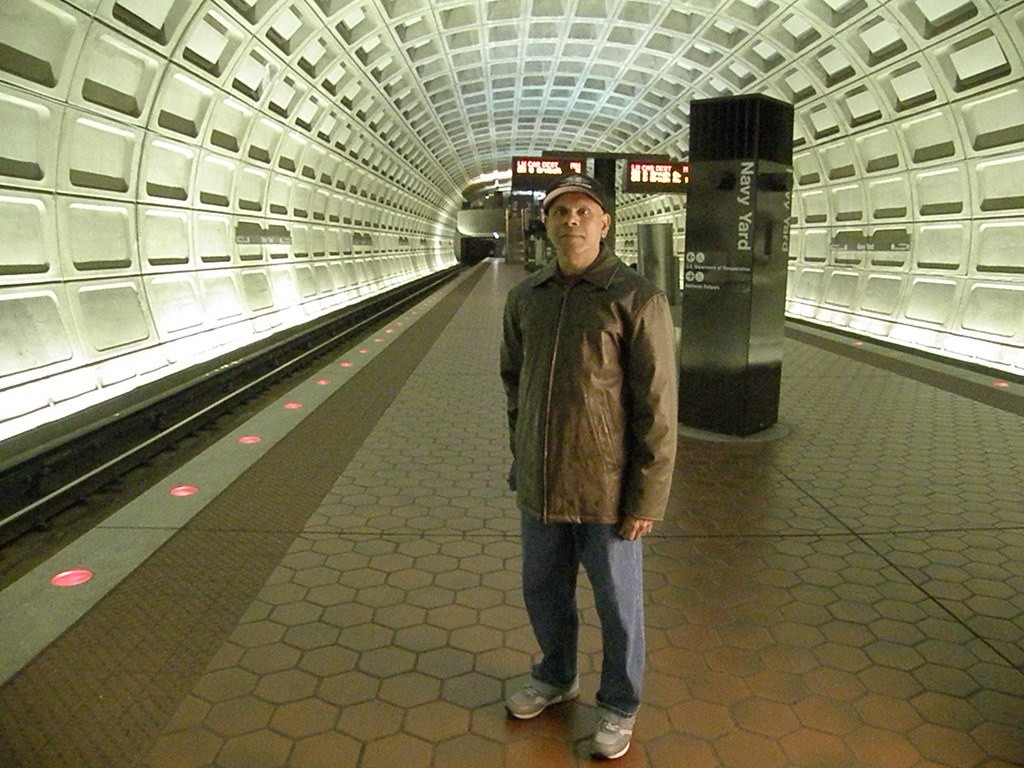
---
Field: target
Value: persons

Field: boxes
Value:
[498,172,679,761]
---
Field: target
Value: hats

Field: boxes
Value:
[543,173,606,214]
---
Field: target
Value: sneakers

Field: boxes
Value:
[590,718,633,759]
[504,668,580,719]
